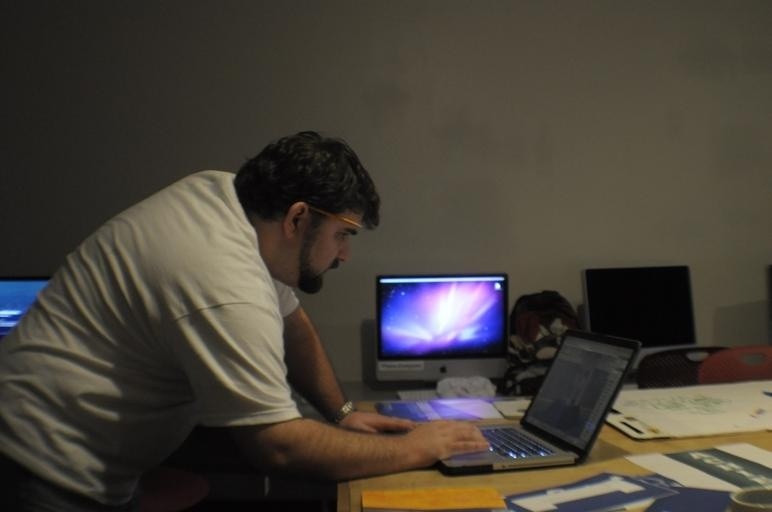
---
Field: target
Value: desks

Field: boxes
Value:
[338,379,772,511]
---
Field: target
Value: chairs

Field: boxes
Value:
[638,346,772,388]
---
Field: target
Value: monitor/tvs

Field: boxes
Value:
[374,272,510,400]
[0,275,52,337]
[581,265,696,370]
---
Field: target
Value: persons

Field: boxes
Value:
[0,128,493,511]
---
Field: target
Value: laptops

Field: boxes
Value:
[433,329,643,474]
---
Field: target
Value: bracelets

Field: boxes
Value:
[331,402,356,424]
